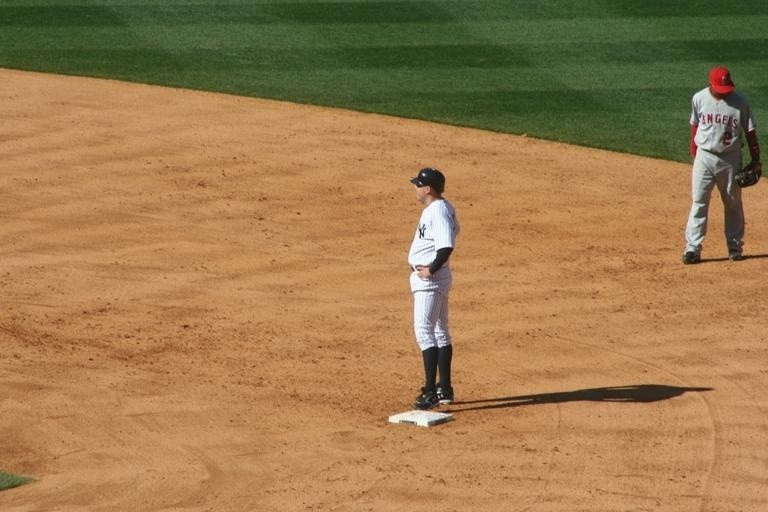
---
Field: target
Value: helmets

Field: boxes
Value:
[409,168,445,187]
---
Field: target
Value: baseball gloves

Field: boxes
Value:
[735,163,761,187]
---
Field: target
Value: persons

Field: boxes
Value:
[682,67,760,264]
[409,169,460,411]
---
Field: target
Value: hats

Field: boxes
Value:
[709,66,735,94]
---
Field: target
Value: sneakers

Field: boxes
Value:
[682,252,700,264]
[413,383,454,409]
[728,250,742,260]
